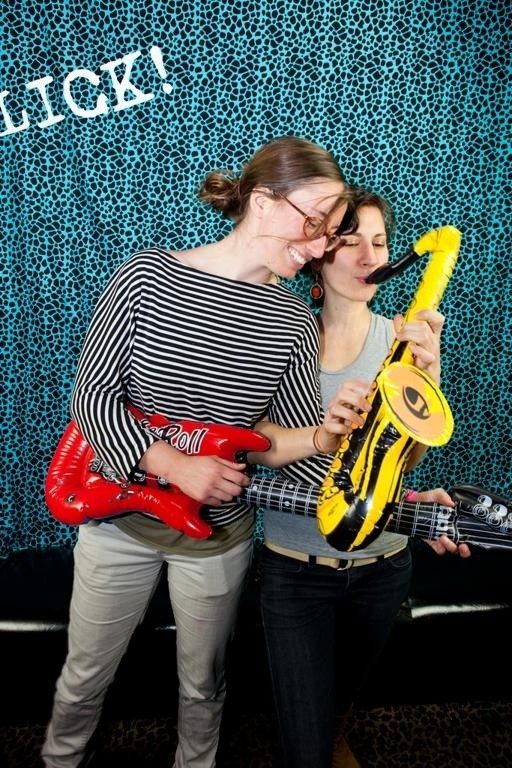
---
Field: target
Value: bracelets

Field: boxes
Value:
[311,426,341,456]
[404,489,417,502]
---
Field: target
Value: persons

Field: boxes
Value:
[33,133,475,767]
[241,184,445,767]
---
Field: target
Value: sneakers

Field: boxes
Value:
[330,713,361,767]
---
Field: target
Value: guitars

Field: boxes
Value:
[41,397,512,564]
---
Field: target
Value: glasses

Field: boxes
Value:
[273,190,341,253]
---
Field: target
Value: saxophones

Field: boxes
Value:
[315,222,464,553]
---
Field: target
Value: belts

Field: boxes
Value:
[262,538,408,571]
